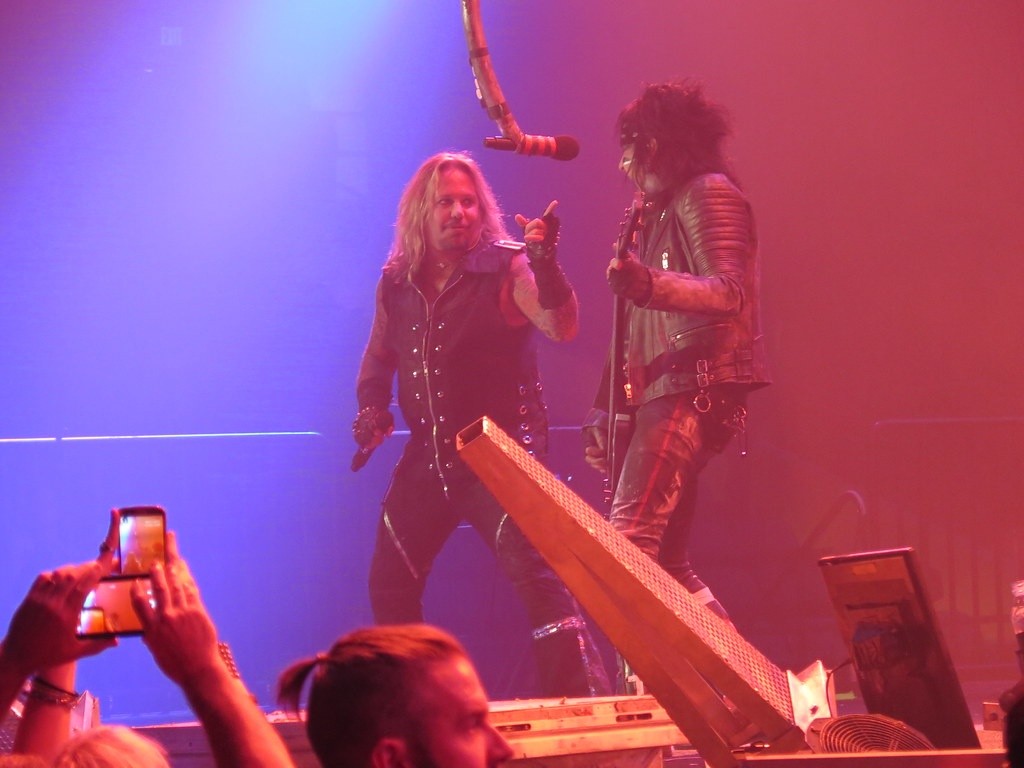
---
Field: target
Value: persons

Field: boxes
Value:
[0,510,294,768]
[279,623,514,768]
[353,152,615,697]
[582,80,773,631]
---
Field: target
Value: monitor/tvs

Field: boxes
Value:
[818,547,983,753]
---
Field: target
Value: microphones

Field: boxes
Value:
[350,410,394,472]
[484,133,580,161]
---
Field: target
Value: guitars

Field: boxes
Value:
[600,195,643,505]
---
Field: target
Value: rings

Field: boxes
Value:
[99,542,116,552]
[44,581,56,592]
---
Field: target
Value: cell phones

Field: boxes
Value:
[115,504,168,576]
[75,575,156,637]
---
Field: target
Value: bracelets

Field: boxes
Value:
[30,677,78,706]
[217,642,240,678]
